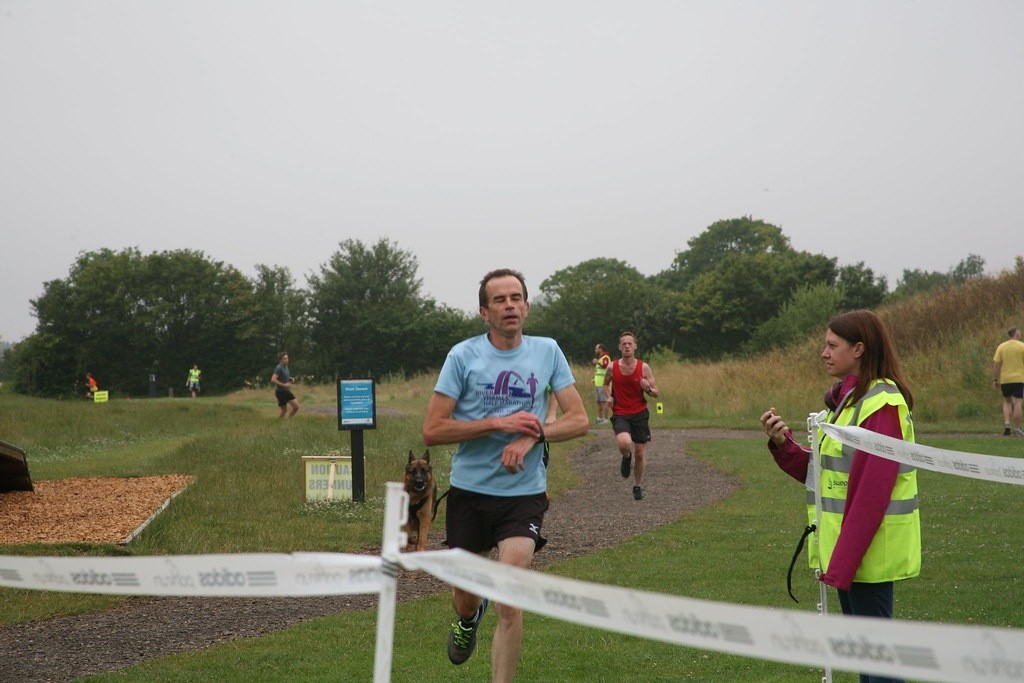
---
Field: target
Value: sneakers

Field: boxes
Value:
[632,485,643,500]
[620,452,631,477]
[596,417,603,422]
[1003,425,1011,436]
[1014,428,1024,436]
[599,419,608,424]
[449,597,488,664]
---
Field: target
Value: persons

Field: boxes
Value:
[185,365,202,399]
[85,373,98,400]
[423,268,590,683]
[592,344,611,425]
[993,328,1024,437]
[270,352,300,417]
[760,311,922,683]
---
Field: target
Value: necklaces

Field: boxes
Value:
[604,332,659,500]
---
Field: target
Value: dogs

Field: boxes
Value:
[400,448,438,552]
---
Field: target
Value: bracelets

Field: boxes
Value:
[536,428,545,444]
[994,379,998,381]
[646,387,652,394]
